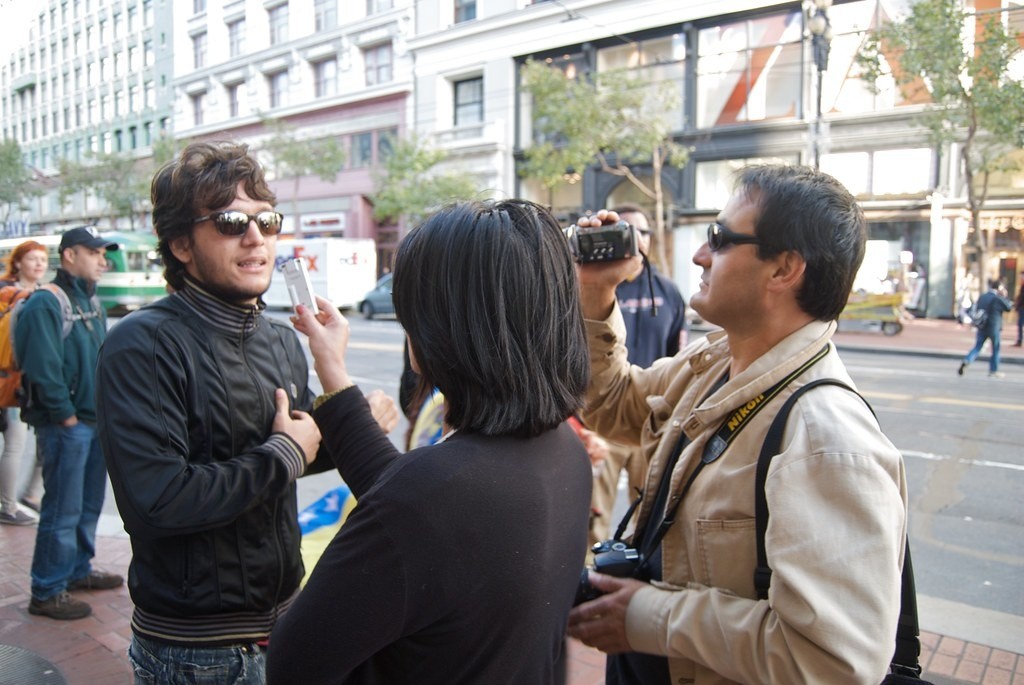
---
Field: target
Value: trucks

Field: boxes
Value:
[260,238,377,310]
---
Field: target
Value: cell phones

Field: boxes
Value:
[998,285,1004,291]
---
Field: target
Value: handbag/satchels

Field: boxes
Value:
[971,295,998,330]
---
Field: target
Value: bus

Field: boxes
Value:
[0,231,169,317]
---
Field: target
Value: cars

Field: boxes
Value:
[904,262,928,318]
[358,271,395,321]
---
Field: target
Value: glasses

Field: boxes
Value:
[707,222,764,252]
[191,209,284,237]
[639,228,650,237]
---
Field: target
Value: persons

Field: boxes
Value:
[1010,271,1024,347]
[379,266,390,279]
[0,241,48,525]
[957,280,1012,378]
[566,162,909,685]
[573,205,689,568]
[93,144,399,685]
[266,198,593,685]
[15,225,124,620]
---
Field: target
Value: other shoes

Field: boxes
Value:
[988,371,1005,379]
[958,360,967,375]
[0,508,35,525]
[20,495,43,513]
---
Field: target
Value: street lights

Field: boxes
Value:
[807,0,834,170]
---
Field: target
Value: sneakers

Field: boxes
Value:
[28,589,91,620]
[66,569,123,592]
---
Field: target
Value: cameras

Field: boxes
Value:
[571,539,653,609]
[561,221,637,263]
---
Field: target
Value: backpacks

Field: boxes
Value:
[0,285,102,431]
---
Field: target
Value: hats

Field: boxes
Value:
[59,225,120,252]
